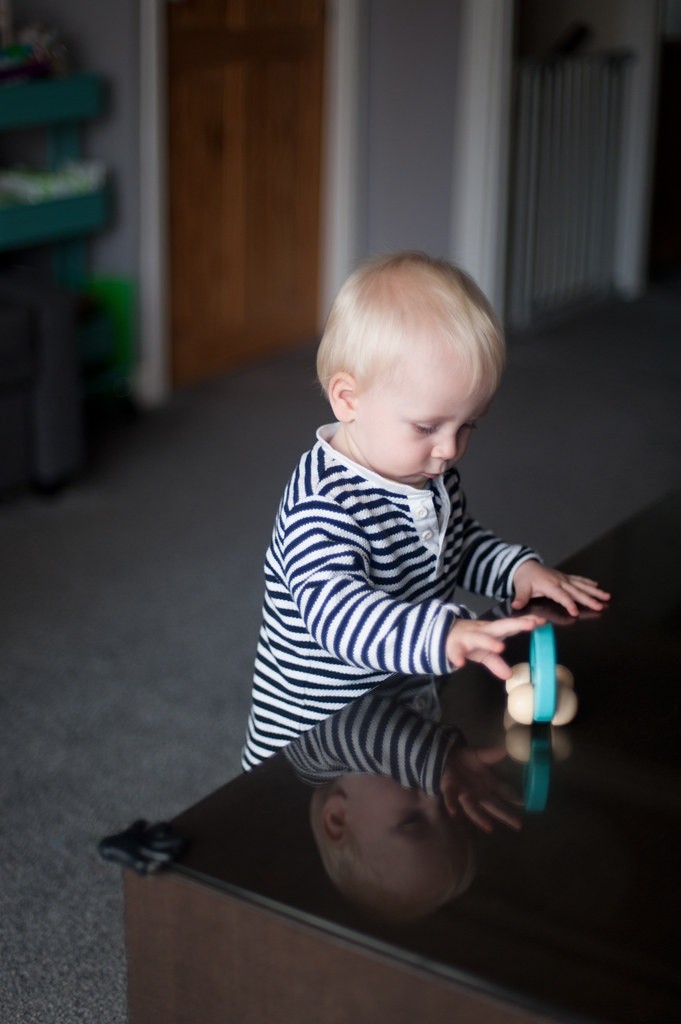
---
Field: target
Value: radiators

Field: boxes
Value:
[509,54,620,328]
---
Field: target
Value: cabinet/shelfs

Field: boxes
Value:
[0,70,117,288]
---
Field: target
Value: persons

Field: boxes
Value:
[239,251,611,776]
[283,671,526,916]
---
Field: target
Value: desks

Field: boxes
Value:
[120,487,681,1024]
